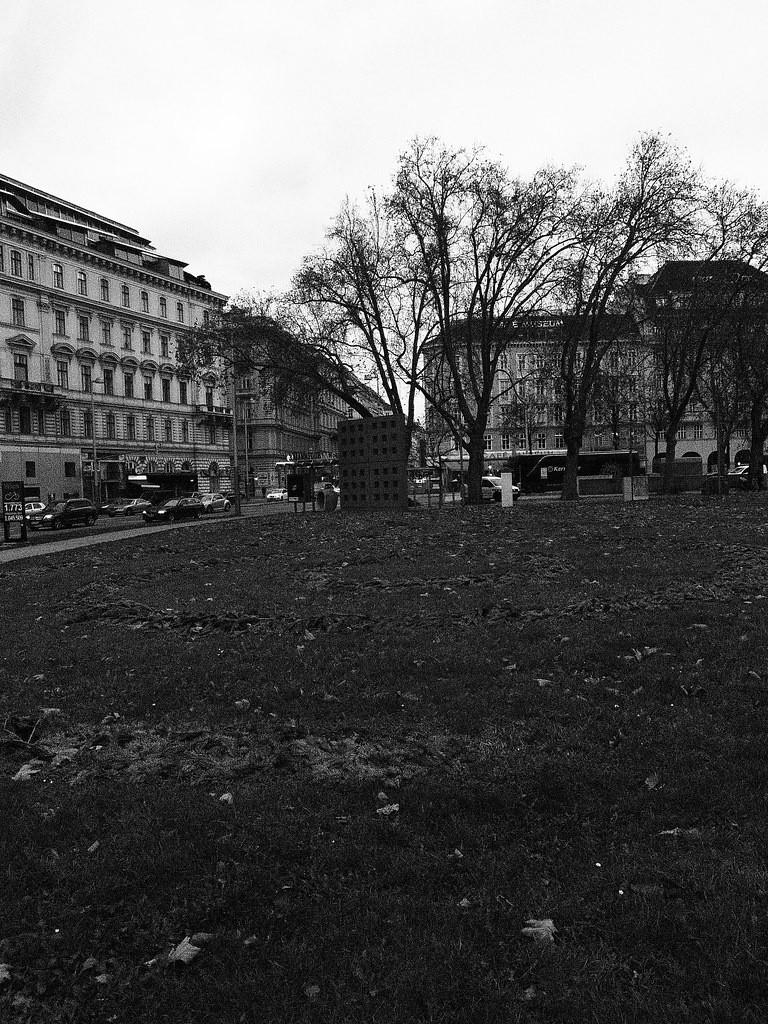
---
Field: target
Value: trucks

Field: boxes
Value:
[523,450,641,496]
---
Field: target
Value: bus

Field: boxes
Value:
[405,467,448,496]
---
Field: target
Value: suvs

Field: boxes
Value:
[26,497,99,531]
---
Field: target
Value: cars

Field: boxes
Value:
[460,476,520,502]
[108,498,154,518]
[142,497,206,522]
[266,488,288,502]
[219,491,236,507]
[312,482,340,500]
[197,493,232,514]
[97,498,118,517]
[24,501,46,517]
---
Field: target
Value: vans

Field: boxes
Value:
[727,465,768,475]
[139,490,203,508]
